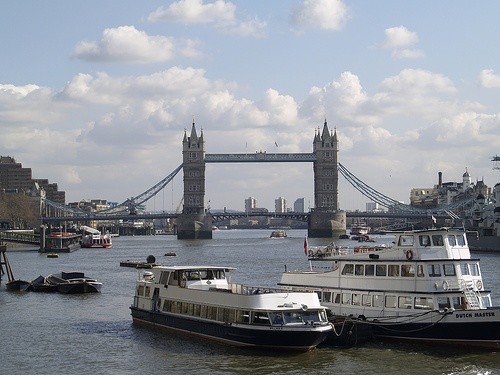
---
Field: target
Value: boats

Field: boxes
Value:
[129,265,333,350]
[270,231,287,239]
[307,244,385,260]
[29,275,57,293]
[219,225,227,230]
[120,260,161,269]
[212,225,217,230]
[7,277,32,294]
[337,222,387,243]
[164,252,176,256]
[48,254,58,258]
[45,271,103,295]
[276,227,500,349]
[81,235,112,249]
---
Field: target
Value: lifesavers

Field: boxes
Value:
[476,280,482,290]
[442,281,449,291]
[406,250,413,260]
[308,249,314,256]
[317,250,323,255]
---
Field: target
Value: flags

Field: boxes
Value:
[304,237,308,256]
